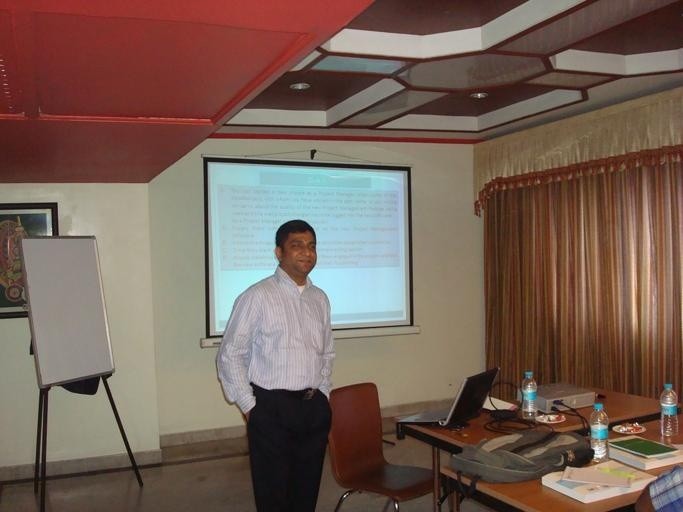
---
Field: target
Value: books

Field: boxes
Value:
[608,436,679,457]
[607,434,683,471]
[542,459,658,503]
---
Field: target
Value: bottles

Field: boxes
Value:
[660,384,678,437]
[589,403,609,462]
[522,371,538,419]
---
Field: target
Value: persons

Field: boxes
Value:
[216,220,335,511]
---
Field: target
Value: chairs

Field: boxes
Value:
[328,382,454,511]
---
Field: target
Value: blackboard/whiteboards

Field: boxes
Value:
[18,236,116,389]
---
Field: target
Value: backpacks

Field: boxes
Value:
[448,429,594,498]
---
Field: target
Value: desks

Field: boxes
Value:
[396,385,682,511]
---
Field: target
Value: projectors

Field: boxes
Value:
[517,382,596,414]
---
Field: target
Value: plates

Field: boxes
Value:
[535,415,566,424]
[612,424,646,435]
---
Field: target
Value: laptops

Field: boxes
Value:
[395,367,501,427]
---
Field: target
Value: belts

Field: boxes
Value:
[290,389,319,401]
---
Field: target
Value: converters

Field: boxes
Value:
[491,410,517,420]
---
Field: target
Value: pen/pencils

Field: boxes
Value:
[597,466,636,479]
